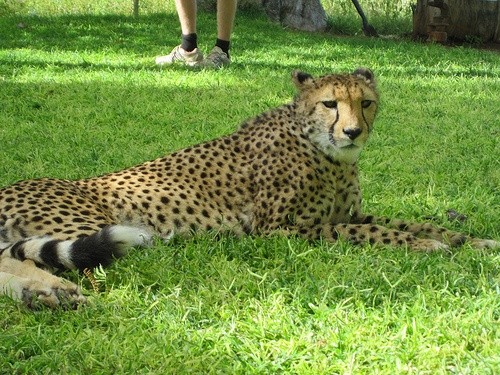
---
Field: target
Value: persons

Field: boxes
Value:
[154,0,237,67]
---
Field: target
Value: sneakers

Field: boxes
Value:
[155,46,201,68]
[203,46,230,67]
